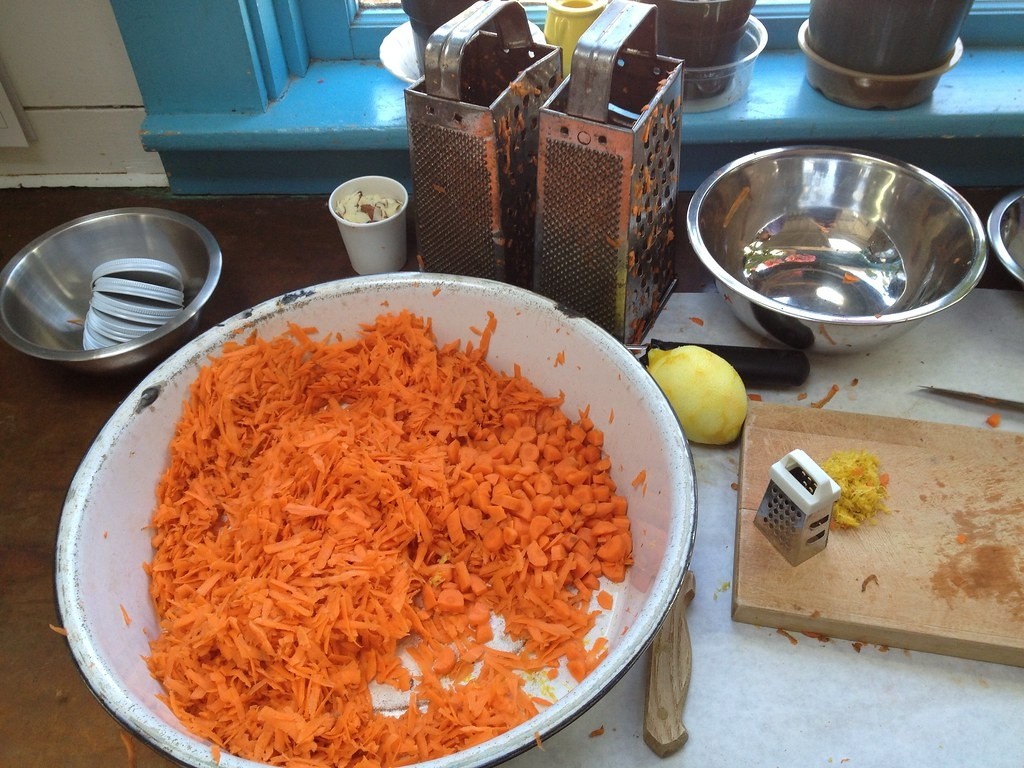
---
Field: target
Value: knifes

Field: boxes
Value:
[918,385,1024,413]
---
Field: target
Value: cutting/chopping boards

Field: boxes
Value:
[731,401,1024,667]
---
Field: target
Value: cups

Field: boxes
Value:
[327,175,409,275]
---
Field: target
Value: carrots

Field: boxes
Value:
[144,309,634,768]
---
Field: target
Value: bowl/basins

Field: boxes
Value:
[797,19,964,111]
[0,206,223,378]
[378,18,546,86]
[685,144,989,356]
[986,187,1024,284]
[683,13,768,115]
[52,271,700,768]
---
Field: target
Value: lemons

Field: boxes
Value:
[648,346,748,446]
